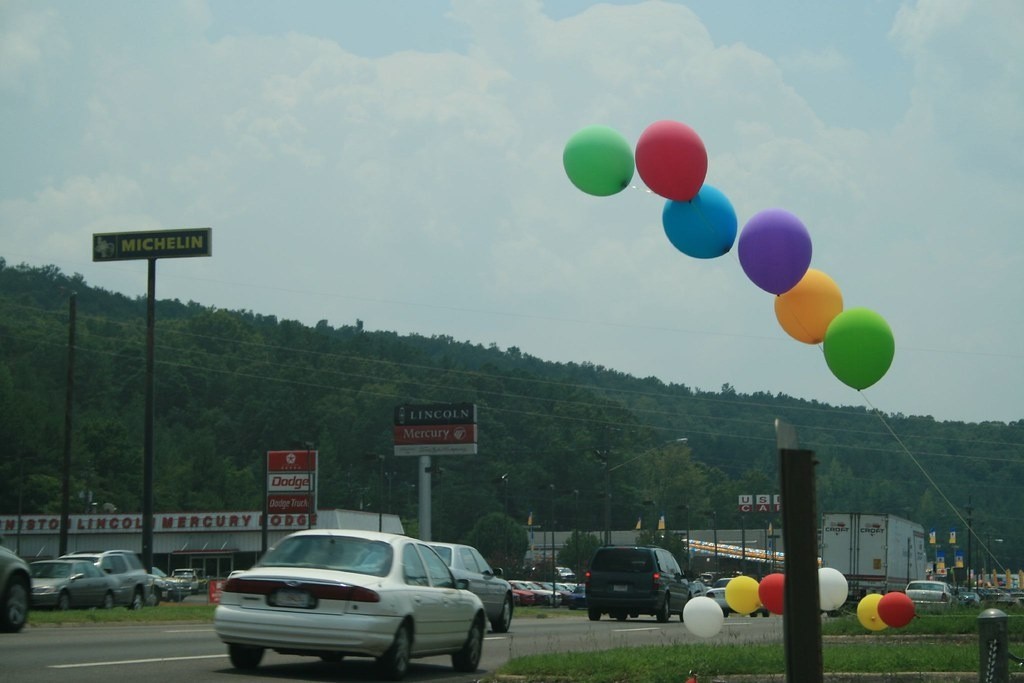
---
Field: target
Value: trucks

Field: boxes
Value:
[819,511,929,599]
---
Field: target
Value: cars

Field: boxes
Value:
[145,566,210,607]
[692,570,771,619]
[422,541,515,634]
[0,545,33,634]
[28,559,117,612]
[905,578,959,615]
[212,527,488,683]
[506,566,588,610]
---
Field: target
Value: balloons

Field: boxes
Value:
[682,596,725,636]
[824,309,895,391]
[818,567,848,611]
[739,209,813,296]
[759,573,784,614]
[662,185,738,260]
[562,124,634,197]
[878,591,916,630]
[775,270,843,344]
[857,593,888,632]
[725,575,762,615]
[635,121,708,202]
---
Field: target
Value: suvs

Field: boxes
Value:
[584,543,693,624]
[49,549,153,611]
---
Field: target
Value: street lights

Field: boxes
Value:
[422,466,445,543]
[677,504,691,570]
[957,586,1024,611]
[987,534,1005,579]
[733,515,746,576]
[702,509,719,579]
[491,474,509,582]
[643,500,657,546]
[291,441,313,530]
[366,452,387,532]
[537,483,557,609]
[564,489,580,587]
[605,436,690,546]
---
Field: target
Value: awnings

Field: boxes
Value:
[172,533,240,554]
